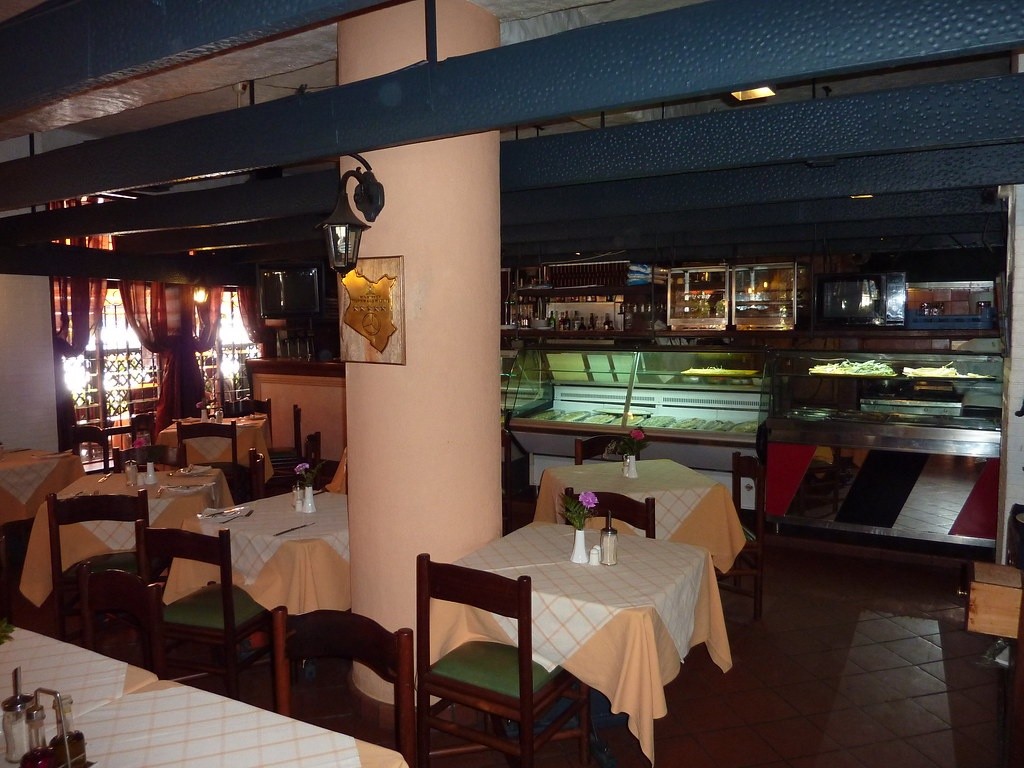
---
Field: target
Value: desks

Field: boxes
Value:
[1,414,747,768]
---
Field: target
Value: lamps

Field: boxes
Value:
[311,167,384,282]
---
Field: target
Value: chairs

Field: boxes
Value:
[47,397,766,768]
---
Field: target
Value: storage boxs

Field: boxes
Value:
[905,288,970,315]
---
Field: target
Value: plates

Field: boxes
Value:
[168,483,206,494]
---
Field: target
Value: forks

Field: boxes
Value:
[216,510,254,524]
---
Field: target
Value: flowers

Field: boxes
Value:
[556,490,599,531]
[614,429,653,456]
[295,460,328,487]
[133,438,162,463]
[196,401,205,410]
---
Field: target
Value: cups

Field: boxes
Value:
[137,474,144,487]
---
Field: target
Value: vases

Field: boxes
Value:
[570,529,589,564]
[146,462,157,484]
[201,410,208,422]
[302,486,316,514]
[628,455,638,479]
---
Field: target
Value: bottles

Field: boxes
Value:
[48,694,87,768]
[1,694,35,764]
[215,408,223,423]
[547,310,614,331]
[20,704,57,767]
[210,417,214,423]
[124,459,139,487]
[622,461,629,477]
[292,485,304,512]
[588,528,619,566]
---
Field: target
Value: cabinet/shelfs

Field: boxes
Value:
[503,344,1005,567]
